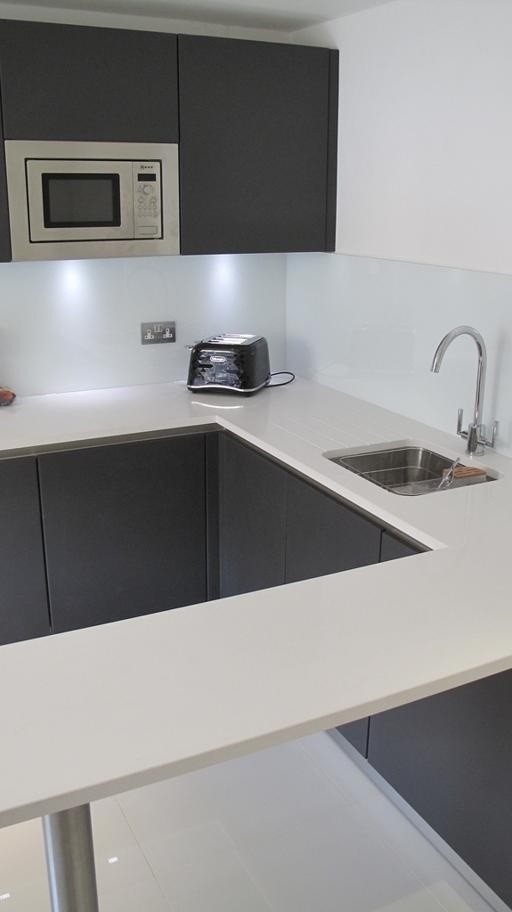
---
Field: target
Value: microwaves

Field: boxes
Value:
[3,139,181,263]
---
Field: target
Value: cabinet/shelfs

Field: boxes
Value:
[219,437,380,598]
[1,19,176,144]
[0,459,50,644]
[366,532,511,912]
[178,36,339,254]
[37,436,207,636]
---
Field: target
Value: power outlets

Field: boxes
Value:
[141,323,175,345]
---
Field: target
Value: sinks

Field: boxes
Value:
[323,438,505,497]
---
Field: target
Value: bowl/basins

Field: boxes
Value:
[0,391,16,405]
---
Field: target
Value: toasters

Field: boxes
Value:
[184,332,272,396]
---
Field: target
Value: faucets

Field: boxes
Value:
[430,324,485,456]
